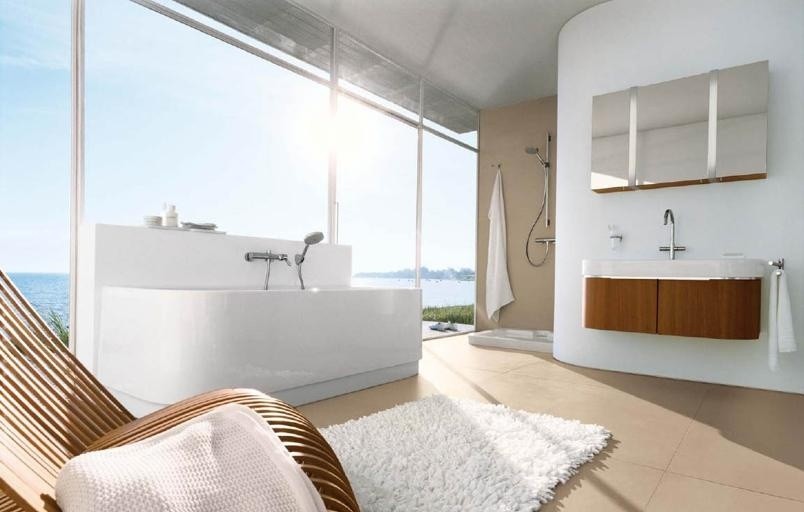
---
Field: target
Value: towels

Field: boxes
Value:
[766,268,799,371]
[484,169,517,324]
[55,401,326,511]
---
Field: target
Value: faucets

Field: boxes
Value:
[246,250,292,290]
[663,209,676,261]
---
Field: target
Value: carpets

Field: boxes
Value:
[314,391,614,511]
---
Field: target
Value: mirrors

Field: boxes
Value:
[589,59,773,193]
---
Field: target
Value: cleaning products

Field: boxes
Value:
[165,204,179,227]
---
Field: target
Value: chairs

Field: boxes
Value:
[0,270,358,511]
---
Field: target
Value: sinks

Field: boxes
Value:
[580,259,769,279]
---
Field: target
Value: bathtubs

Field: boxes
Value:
[100,287,423,402]
